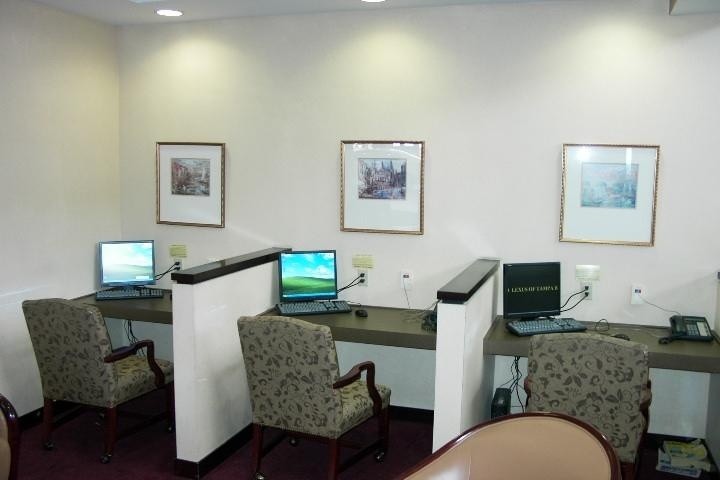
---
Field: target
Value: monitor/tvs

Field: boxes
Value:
[278,250,337,302]
[503,261,561,320]
[99,240,156,289]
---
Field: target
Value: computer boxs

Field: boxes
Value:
[491,388,511,420]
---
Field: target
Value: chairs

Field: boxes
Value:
[237,316,393,480]
[397,413,625,480]
[22,298,174,465]
[0,395,20,479]
[526,331,653,480]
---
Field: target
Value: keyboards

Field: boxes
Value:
[506,317,587,337]
[96,289,164,300]
[275,300,352,316]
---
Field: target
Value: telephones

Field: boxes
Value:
[668,315,713,342]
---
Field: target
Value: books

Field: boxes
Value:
[662,440,692,468]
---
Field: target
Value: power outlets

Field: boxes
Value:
[580,282,593,301]
[357,269,368,287]
[173,259,181,271]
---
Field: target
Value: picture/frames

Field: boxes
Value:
[559,142,663,247]
[337,138,427,236]
[156,141,228,229]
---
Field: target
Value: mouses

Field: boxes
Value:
[613,333,630,341]
[355,310,368,317]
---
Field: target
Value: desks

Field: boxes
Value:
[258,304,436,350]
[483,313,720,374]
[71,287,173,326]
